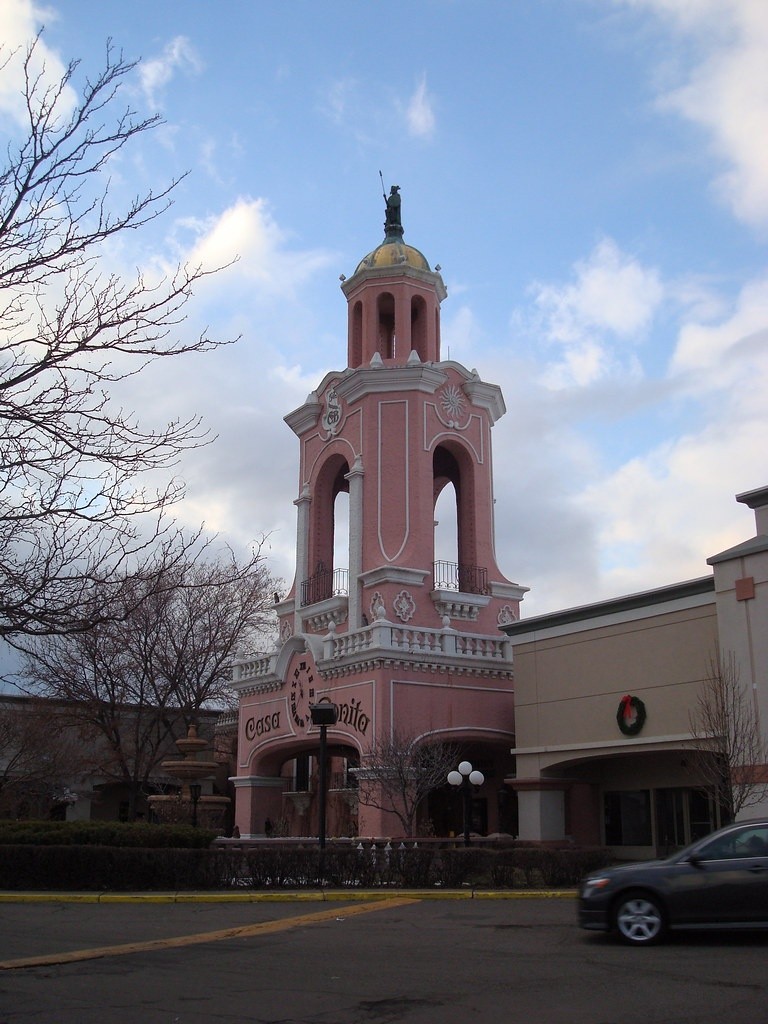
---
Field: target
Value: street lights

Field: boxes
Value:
[447,762,485,849]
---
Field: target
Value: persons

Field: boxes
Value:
[265,817,275,838]
[233,825,241,839]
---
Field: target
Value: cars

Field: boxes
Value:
[575,816,768,947]
